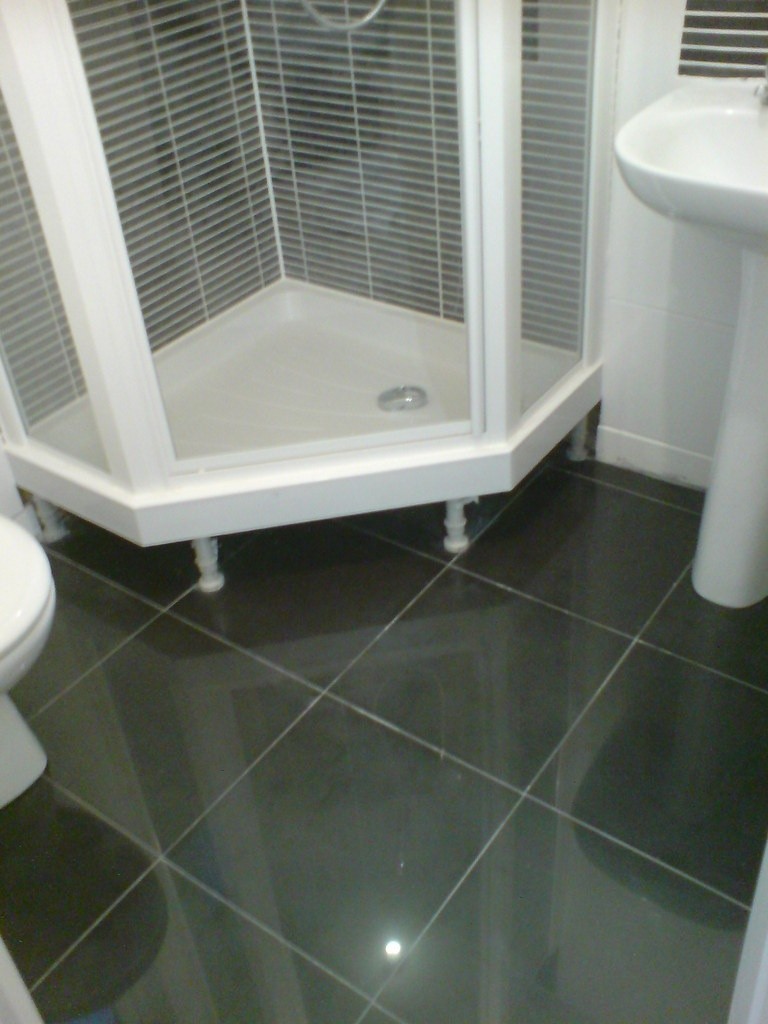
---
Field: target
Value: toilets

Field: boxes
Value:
[0,518,57,808]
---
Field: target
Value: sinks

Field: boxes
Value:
[611,84,767,237]
